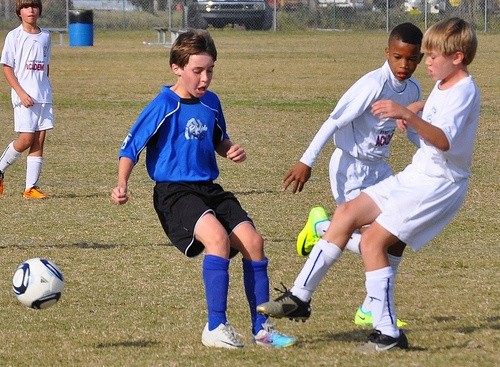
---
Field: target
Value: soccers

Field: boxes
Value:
[12,258,65,311]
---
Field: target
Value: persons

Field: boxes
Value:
[253,16,481,356]
[0,0,61,199]
[283,22,425,327]
[111,32,297,348]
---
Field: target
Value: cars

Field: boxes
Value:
[180,0,272,31]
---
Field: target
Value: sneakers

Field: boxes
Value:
[23,186,51,199]
[354,306,407,328]
[253,322,299,348]
[256,282,311,322]
[0,169,4,194]
[297,206,327,256]
[201,322,243,348]
[357,330,408,356]
